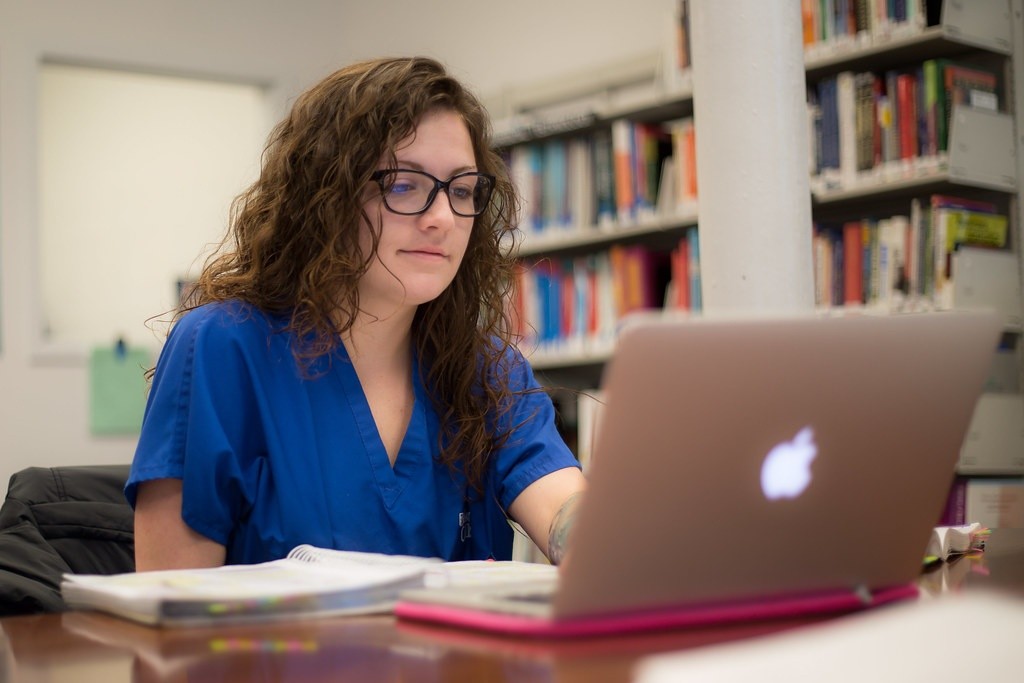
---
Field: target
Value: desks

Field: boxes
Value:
[0,614,856,683]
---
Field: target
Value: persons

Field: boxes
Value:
[125,58,589,573]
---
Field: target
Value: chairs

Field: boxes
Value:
[0,465,134,614]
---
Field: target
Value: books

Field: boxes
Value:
[481,0,1024,548]
[59,544,444,630]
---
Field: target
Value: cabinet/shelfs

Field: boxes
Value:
[483,0,1024,477]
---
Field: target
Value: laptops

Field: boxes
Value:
[402,308,1000,623]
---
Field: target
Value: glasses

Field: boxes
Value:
[369,169,496,216]
